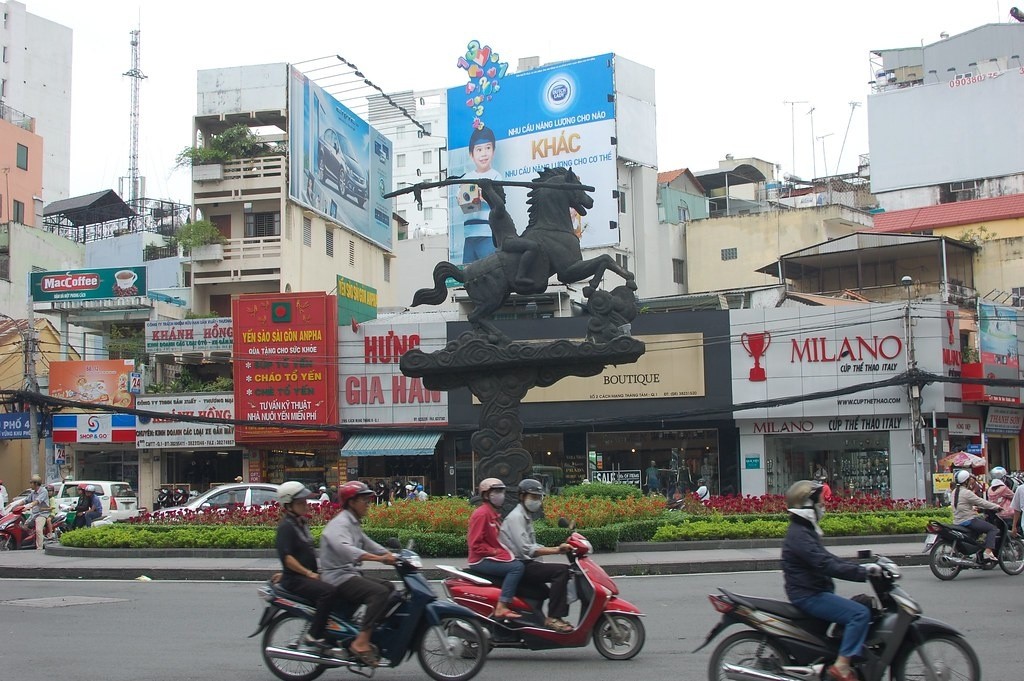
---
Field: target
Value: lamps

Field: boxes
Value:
[611,136,617,144]
[420,97,447,104]
[608,94,614,102]
[417,169,446,176]
[418,131,446,138]
[658,432,664,439]
[692,430,697,438]
[418,202,447,211]
[613,190,619,199]
[607,59,613,67]
[680,431,686,439]
[421,244,449,251]
[610,221,618,229]
[983,288,1024,307]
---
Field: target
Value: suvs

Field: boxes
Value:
[49,481,139,524]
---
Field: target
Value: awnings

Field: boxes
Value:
[340,431,445,457]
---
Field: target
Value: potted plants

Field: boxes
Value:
[173,221,229,261]
[170,123,287,180]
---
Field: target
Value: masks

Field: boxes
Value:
[524,497,542,512]
[814,503,825,521]
[489,491,505,507]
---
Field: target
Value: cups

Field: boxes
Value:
[115,270,138,291]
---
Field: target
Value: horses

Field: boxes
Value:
[407,166,637,344]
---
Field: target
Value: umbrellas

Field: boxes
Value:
[938,450,987,469]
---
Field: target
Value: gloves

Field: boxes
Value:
[859,563,882,577]
[997,504,1001,512]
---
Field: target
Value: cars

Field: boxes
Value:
[148,483,322,525]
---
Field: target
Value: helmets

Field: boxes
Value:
[786,480,824,508]
[819,475,827,480]
[330,485,336,491]
[405,485,423,492]
[697,478,706,483]
[479,478,506,497]
[518,479,546,497]
[319,486,326,492]
[0,480,2,485]
[278,481,312,506]
[30,476,95,493]
[954,467,1006,484]
[339,481,373,504]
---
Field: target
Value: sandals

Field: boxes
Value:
[545,617,573,632]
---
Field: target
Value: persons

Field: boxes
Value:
[275,481,339,649]
[478,178,539,284]
[235,476,243,483]
[944,466,1024,562]
[700,457,713,494]
[814,462,828,482]
[581,477,591,485]
[498,479,575,632]
[320,481,396,668]
[466,477,525,619]
[780,480,882,681]
[675,459,693,491]
[319,485,429,502]
[0,474,103,550]
[643,460,661,493]
[647,488,659,498]
[456,126,503,264]
[691,479,711,506]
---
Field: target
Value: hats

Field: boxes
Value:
[235,476,243,481]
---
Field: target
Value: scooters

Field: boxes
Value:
[435,518,647,660]
[0,505,113,551]
[247,537,487,681]
[922,507,1024,581]
[155,485,188,509]
[691,550,982,681]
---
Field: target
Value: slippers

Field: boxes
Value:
[495,610,522,619]
[349,647,378,669]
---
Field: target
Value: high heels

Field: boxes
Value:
[983,550,999,561]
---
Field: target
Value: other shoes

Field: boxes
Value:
[306,632,333,648]
[827,665,859,681]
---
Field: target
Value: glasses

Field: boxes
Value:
[353,498,373,505]
